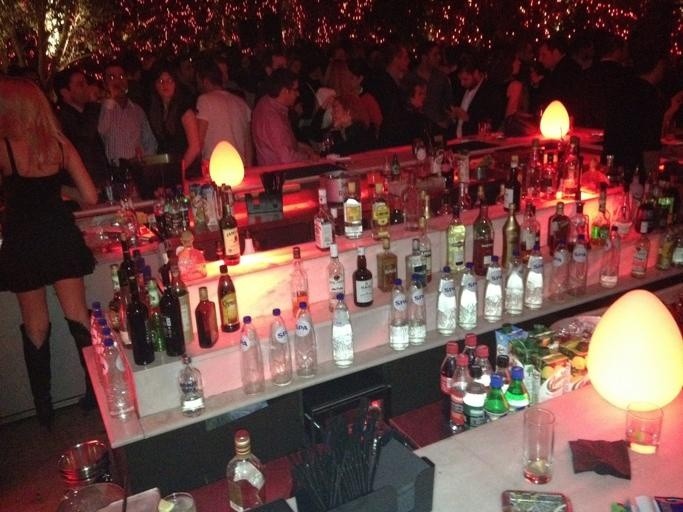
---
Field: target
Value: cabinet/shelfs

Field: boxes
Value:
[0,197,170,427]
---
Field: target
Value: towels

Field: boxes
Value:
[568,439,632,482]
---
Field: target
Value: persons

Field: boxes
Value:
[251,68,321,165]
[146,64,202,182]
[549,37,607,127]
[408,41,455,135]
[311,93,367,156]
[349,73,383,135]
[27,19,368,89]
[530,37,568,112]
[195,61,253,176]
[602,39,628,80]
[399,76,440,145]
[98,65,159,169]
[500,49,534,121]
[52,68,110,188]
[447,64,508,138]
[517,41,533,74]
[663,90,683,130]
[368,45,410,138]
[610,46,668,172]
[0,76,99,433]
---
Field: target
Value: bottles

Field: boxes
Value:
[548,238,568,304]
[482,255,504,324]
[377,234,398,292]
[401,171,422,231]
[462,334,478,361]
[171,263,194,345]
[293,302,320,378]
[450,354,468,431]
[599,225,619,289]
[503,250,525,316]
[458,184,471,210]
[143,265,155,280]
[217,264,243,332]
[543,154,559,197]
[110,264,122,308]
[629,219,650,280]
[117,269,135,350]
[404,238,426,289]
[146,277,165,354]
[527,144,540,189]
[158,264,186,358]
[505,161,520,212]
[605,154,616,176]
[153,190,168,237]
[523,244,544,310]
[571,201,591,250]
[89,301,102,324]
[242,229,254,256]
[416,141,429,178]
[212,241,224,261]
[171,199,180,236]
[193,285,220,348]
[429,164,445,213]
[330,293,354,369]
[436,189,452,219]
[492,325,524,355]
[446,205,466,272]
[235,316,268,396]
[200,184,220,229]
[219,197,241,266]
[562,143,582,195]
[502,202,520,269]
[406,273,428,347]
[546,202,570,256]
[671,221,682,267]
[536,208,540,257]
[440,150,455,187]
[101,338,134,424]
[471,204,495,275]
[342,181,363,239]
[178,354,207,418]
[494,183,504,206]
[419,187,432,219]
[163,196,171,233]
[476,165,489,181]
[177,229,207,283]
[126,276,155,366]
[289,246,313,317]
[268,307,292,386]
[521,202,534,263]
[434,266,457,337]
[164,355,165,357]
[570,232,586,297]
[381,155,391,177]
[131,248,141,258]
[473,185,486,206]
[494,355,510,384]
[614,184,632,237]
[99,326,113,340]
[635,176,655,235]
[529,324,552,340]
[485,373,508,421]
[627,165,642,196]
[461,363,486,431]
[370,182,391,240]
[504,367,530,412]
[120,240,137,301]
[417,216,432,284]
[122,196,139,230]
[459,262,478,331]
[314,187,335,250]
[90,310,103,341]
[654,213,676,272]
[664,173,680,223]
[474,345,492,378]
[97,318,108,342]
[656,189,669,229]
[226,430,269,512]
[391,154,400,177]
[439,341,458,430]
[188,185,206,228]
[590,200,609,246]
[328,242,347,311]
[351,246,374,307]
[387,278,409,352]
[583,158,606,191]
[136,255,147,304]
[176,188,189,230]
[531,354,572,402]
[218,184,233,215]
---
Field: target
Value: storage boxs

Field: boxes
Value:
[297,440,433,512]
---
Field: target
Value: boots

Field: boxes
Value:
[19,322,55,435]
[64,310,102,410]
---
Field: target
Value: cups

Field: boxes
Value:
[521,410,556,484]
[627,407,663,456]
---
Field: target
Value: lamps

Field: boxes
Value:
[538,101,570,139]
[587,290,682,411]
[209,139,244,188]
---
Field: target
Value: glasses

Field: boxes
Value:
[105,74,127,81]
[159,79,173,84]
